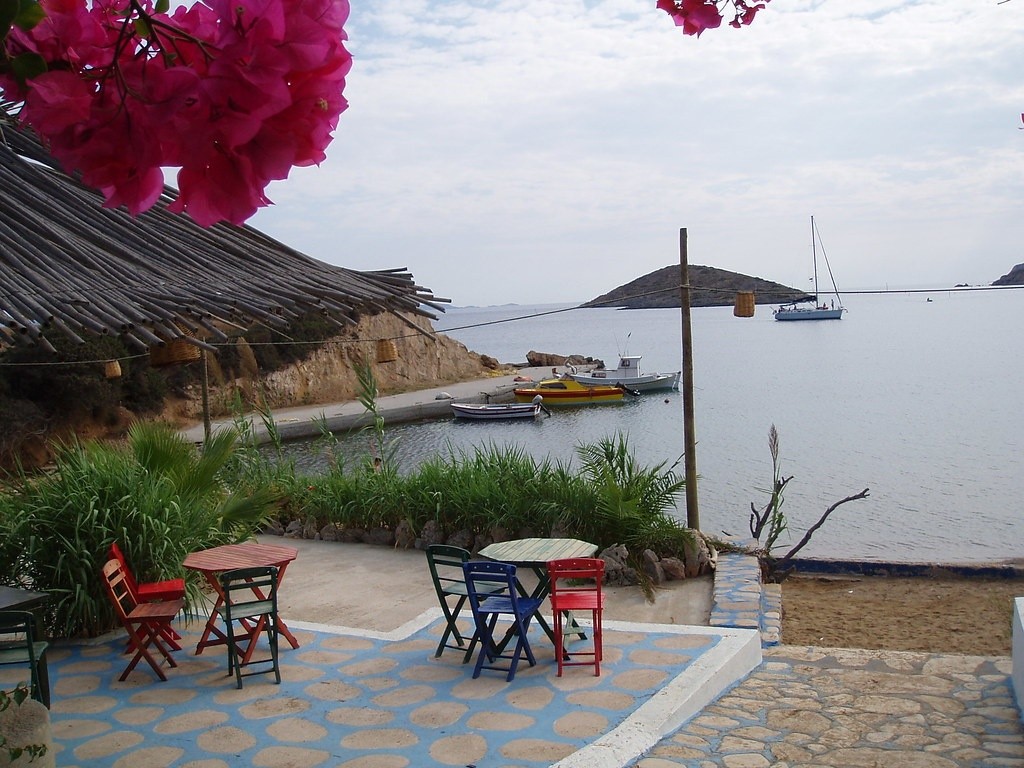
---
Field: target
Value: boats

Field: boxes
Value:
[451,394,550,423]
[552,331,681,395]
[513,371,623,408]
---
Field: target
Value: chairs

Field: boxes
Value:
[546,558,606,678]
[99,559,185,682]
[216,566,282,690]
[0,611,49,712]
[461,561,543,683]
[425,544,509,665]
[107,542,186,655]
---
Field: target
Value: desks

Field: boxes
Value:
[0,586,51,711]
[477,537,600,660]
[182,543,300,668]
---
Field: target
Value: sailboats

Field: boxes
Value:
[769,214,849,321]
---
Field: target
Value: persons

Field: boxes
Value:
[831,299,834,310]
[794,304,796,310]
